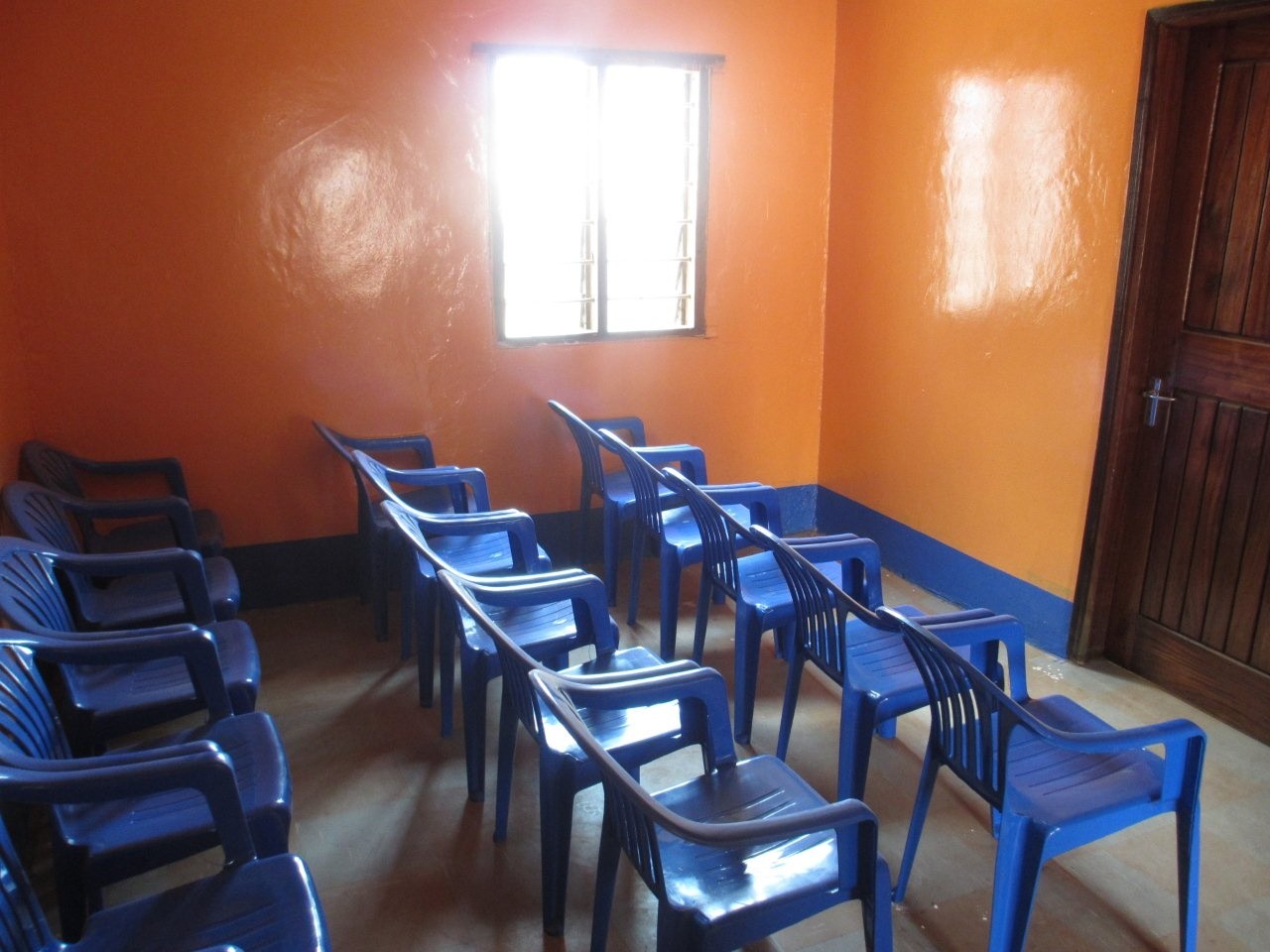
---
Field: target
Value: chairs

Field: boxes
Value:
[0,438,330,952]
[314,399,1212,952]
